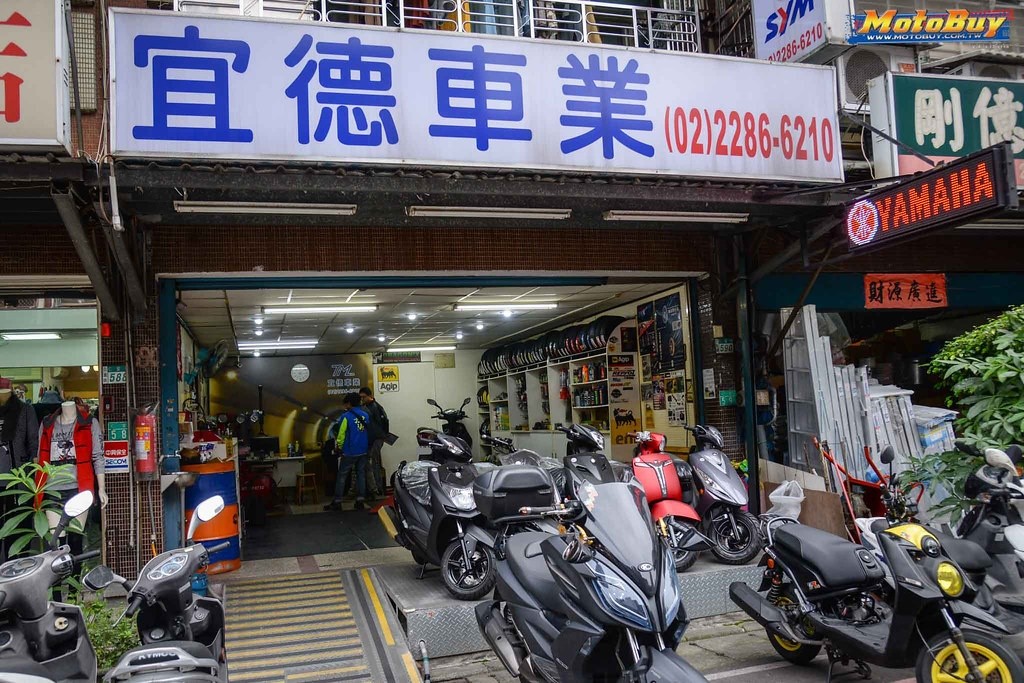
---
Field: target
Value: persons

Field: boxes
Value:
[323,392,371,511]
[0,388,40,560]
[33,401,109,605]
[360,387,387,500]
[323,424,351,496]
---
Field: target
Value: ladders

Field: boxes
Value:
[782,305,956,497]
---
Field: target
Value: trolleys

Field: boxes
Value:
[813,436,927,544]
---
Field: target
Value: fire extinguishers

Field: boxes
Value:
[134,402,157,472]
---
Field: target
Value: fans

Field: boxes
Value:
[189,339,230,392]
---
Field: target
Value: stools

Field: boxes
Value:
[297,474,319,506]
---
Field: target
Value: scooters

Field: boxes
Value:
[81,495,235,683]
[727,444,1024,682]
[374,397,762,683]
[0,489,100,683]
[854,442,1024,663]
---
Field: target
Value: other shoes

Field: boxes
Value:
[324,506,335,512]
[354,503,364,509]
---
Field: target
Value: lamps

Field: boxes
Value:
[602,209,750,224]
[386,346,457,353]
[0,331,63,341]
[237,339,319,351]
[453,303,559,311]
[173,199,356,216]
[407,206,572,220]
[261,305,379,315]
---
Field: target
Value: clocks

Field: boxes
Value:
[290,363,310,382]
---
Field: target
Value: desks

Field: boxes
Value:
[274,455,306,487]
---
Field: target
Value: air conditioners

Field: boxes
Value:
[970,58,1024,80]
[833,42,916,113]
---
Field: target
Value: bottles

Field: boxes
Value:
[572,360,608,406]
[295,441,300,452]
[287,443,293,457]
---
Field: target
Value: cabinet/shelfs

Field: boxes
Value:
[479,318,641,461]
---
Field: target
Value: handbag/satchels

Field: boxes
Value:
[367,427,375,450]
[764,481,804,530]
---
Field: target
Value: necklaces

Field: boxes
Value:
[59,413,77,445]
